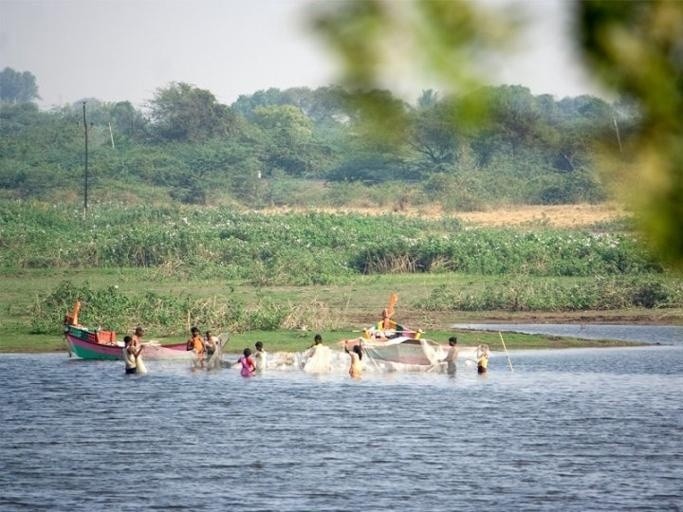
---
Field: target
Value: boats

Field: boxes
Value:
[335,308,480,368]
[60,301,230,362]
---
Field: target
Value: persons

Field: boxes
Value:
[303,335,330,372]
[203,331,218,362]
[254,341,267,372]
[237,348,257,377]
[187,326,207,370]
[123,336,145,374]
[443,336,459,375]
[129,326,148,374]
[477,343,489,374]
[345,339,363,378]
